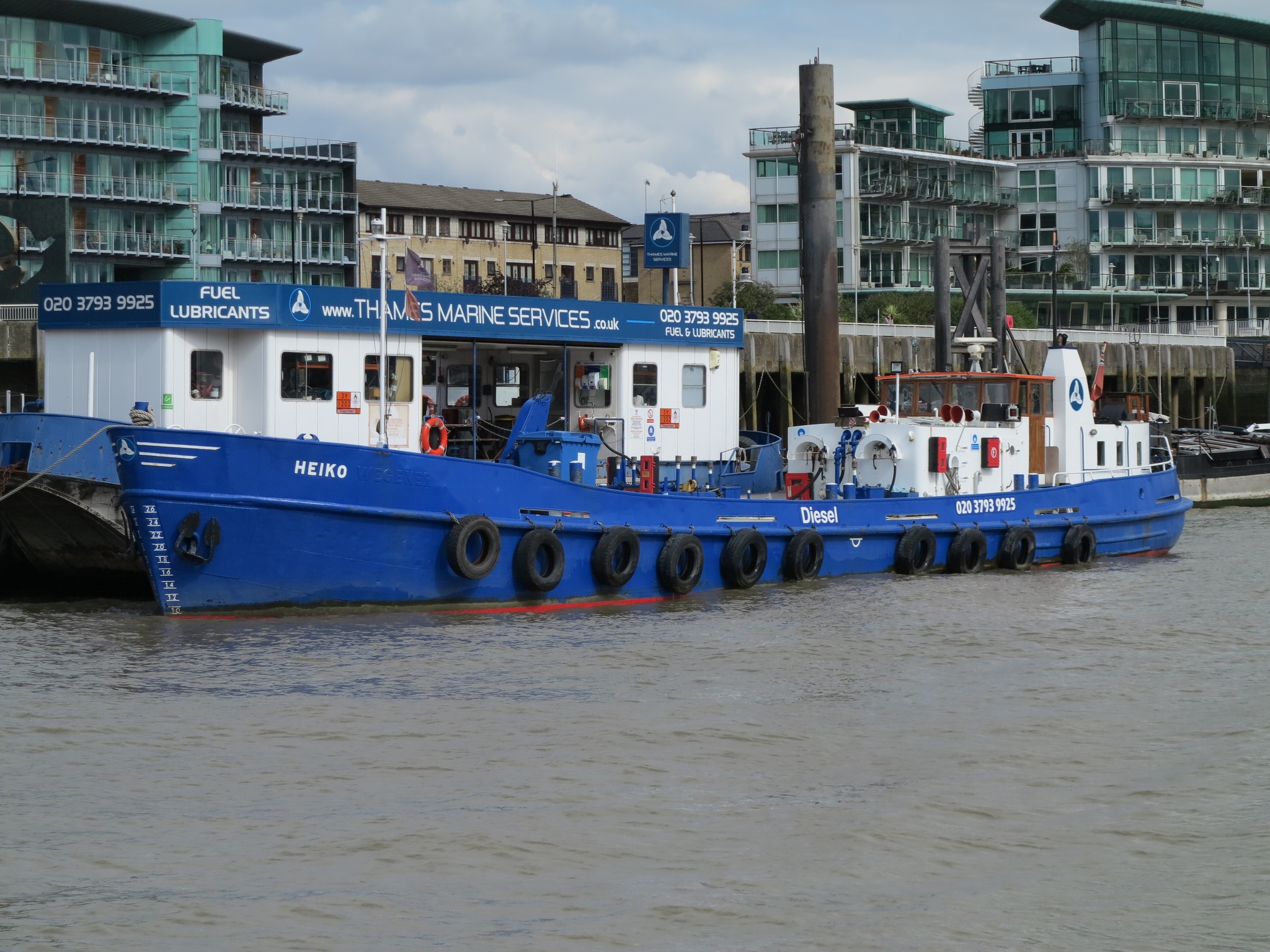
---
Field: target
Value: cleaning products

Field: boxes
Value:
[575,410,589,431]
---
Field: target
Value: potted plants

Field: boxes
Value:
[99,126,108,141]
[257,247,269,258]
[1133,273,1143,290]
[1005,236,1090,291]
[143,238,186,256]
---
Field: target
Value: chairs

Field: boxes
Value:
[1188,278,1203,289]
[1206,189,1232,202]
[1209,105,1251,119]
[767,128,844,145]
[1109,143,1132,156]
[101,183,125,197]
[1184,144,1196,158]
[1110,188,1138,200]
[1208,278,1217,288]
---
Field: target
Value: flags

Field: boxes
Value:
[406,288,422,322]
[1090,347,1104,418]
[405,247,434,292]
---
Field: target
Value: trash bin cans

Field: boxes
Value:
[515,431,603,488]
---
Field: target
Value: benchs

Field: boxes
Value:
[1134,234,1147,242]
[1214,235,1231,246]
[448,436,502,444]
[1174,235,1191,245]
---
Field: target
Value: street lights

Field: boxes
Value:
[1202,238,1213,325]
[498,220,512,296]
[1106,262,1116,332]
[493,194,573,284]
[688,233,696,294]
[1244,242,1252,326]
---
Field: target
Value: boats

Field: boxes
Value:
[1147,424,1270,509]
[0,208,1194,616]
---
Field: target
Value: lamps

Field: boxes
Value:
[420,235,538,249]
[205,244,213,255]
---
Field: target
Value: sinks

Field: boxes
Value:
[584,415,616,429]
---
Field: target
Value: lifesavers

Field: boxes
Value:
[948,528,988,575]
[422,394,435,416]
[514,529,566,593]
[455,395,469,407]
[446,515,502,581]
[1000,525,1036,572]
[658,533,704,594]
[896,525,937,575]
[723,528,768,589]
[786,528,825,581]
[1063,524,1097,564]
[422,416,448,455]
[593,525,641,588]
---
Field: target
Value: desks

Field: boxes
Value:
[1144,239,1213,246]
[235,140,261,151]
[1215,108,1224,118]
[442,423,490,459]
[90,242,108,250]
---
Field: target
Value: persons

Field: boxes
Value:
[882,313,894,326]
